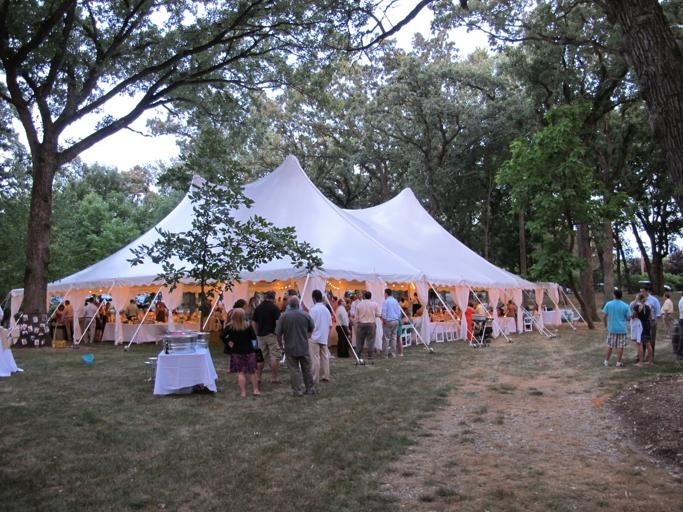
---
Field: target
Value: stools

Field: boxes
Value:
[144,357,158,383]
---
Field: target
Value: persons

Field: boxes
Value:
[95,302,110,342]
[82,298,100,345]
[661,293,673,339]
[349,290,363,321]
[251,290,283,386]
[64,300,73,341]
[629,284,661,362]
[380,288,401,360]
[55,304,64,320]
[678,292,683,356]
[126,299,139,320]
[399,297,410,309]
[220,307,262,399]
[335,298,350,359]
[507,299,516,317]
[398,314,402,357]
[308,290,331,382]
[137,305,152,324]
[629,306,644,366]
[467,298,485,315]
[224,299,251,325]
[602,289,632,366]
[275,296,315,398]
[632,293,653,365]
[354,291,378,358]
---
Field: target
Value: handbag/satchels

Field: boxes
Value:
[250,325,264,362]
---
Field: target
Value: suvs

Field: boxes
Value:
[638,281,671,292]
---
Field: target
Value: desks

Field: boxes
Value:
[101,322,178,344]
[427,318,461,341]
[153,346,218,395]
[497,317,517,335]
[541,311,561,324]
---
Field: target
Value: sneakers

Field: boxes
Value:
[616,361,624,368]
[601,359,609,366]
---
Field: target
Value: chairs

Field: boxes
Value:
[435,321,444,342]
[444,318,455,341]
[524,317,532,332]
[400,325,414,347]
